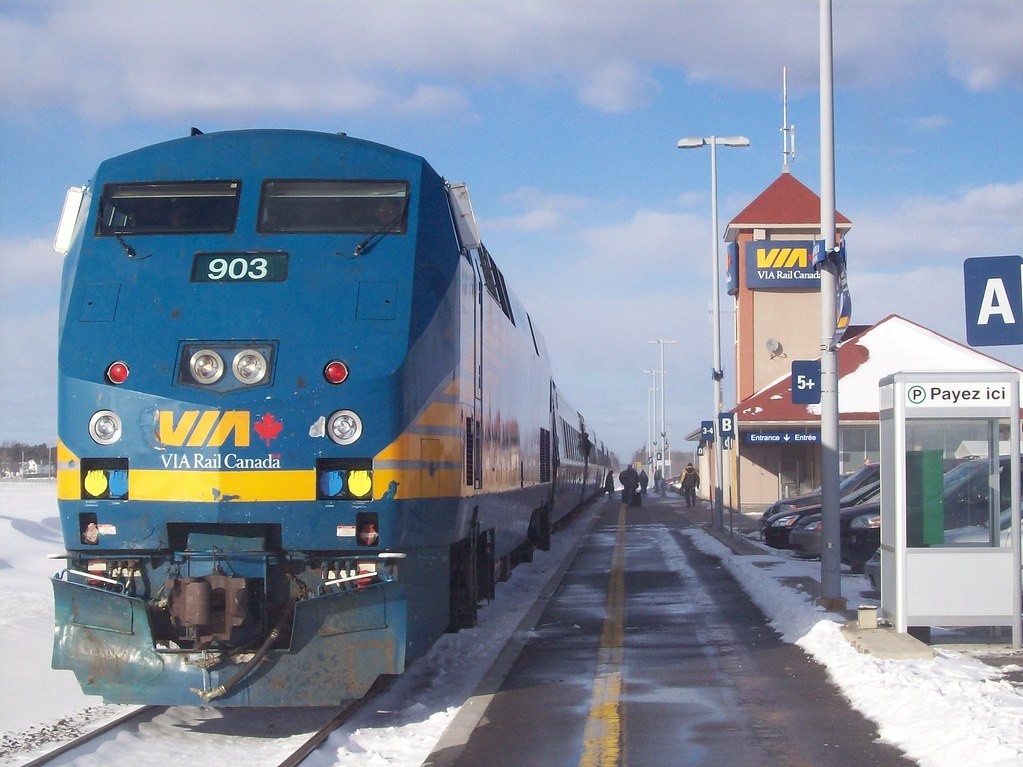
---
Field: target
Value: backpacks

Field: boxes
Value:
[619,471,626,485]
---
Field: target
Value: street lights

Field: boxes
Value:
[643,370,668,473]
[676,134,751,532]
[648,338,677,479]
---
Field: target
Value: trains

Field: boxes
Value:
[48,126,622,707]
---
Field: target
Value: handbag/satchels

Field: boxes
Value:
[680,483,686,495]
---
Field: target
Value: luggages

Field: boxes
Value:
[633,490,642,506]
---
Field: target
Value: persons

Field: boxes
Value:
[681,463,700,507]
[654,467,659,493]
[601,470,614,499]
[863,459,871,467]
[639,470,648,497]
[622,465,639,506]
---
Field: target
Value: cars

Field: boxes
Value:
[759,454,1023,593]
